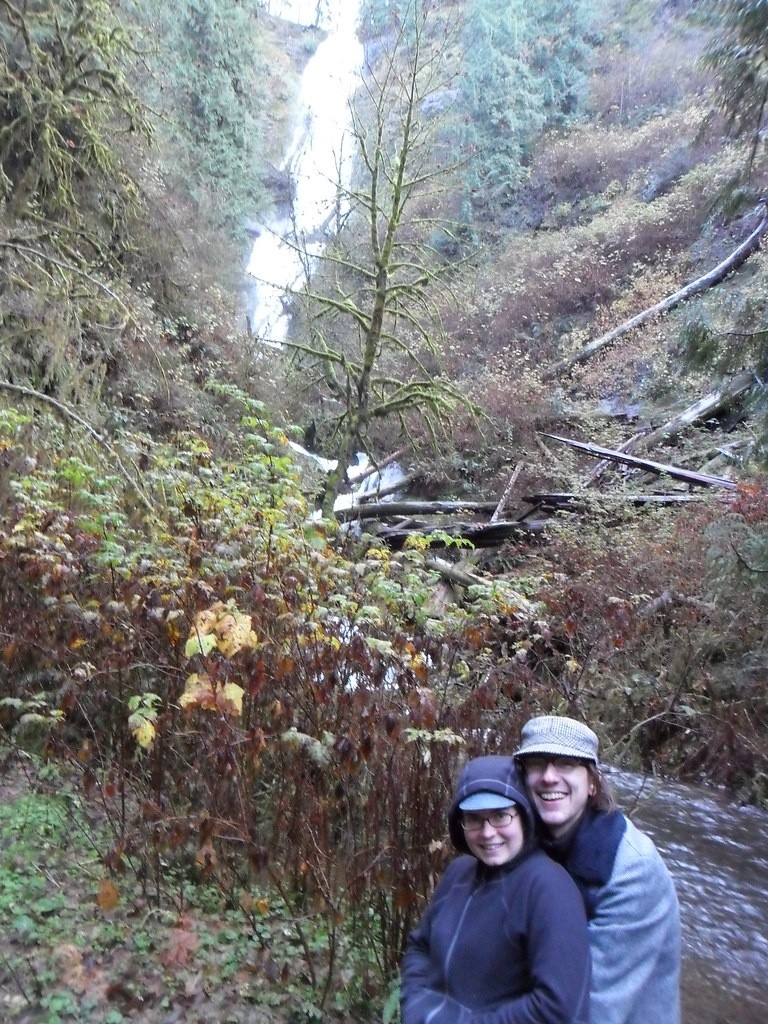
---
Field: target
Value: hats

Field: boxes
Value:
[515,716,599,762]
[459,789,517,811]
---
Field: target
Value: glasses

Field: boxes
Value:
[458,811,522,830]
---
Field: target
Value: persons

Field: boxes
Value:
[402,756,593,1024]
[512,716,681,1024]
